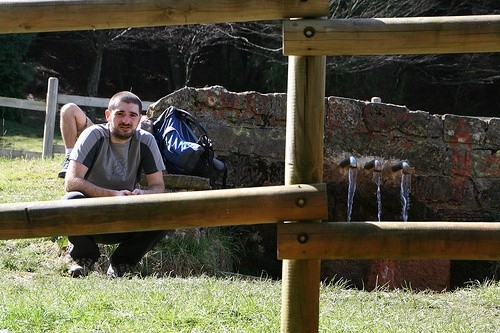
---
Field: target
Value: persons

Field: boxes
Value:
[60,91,171,279]
[59,102,97,178]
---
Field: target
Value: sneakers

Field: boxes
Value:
[107,262,134,281]
[58,154,71,178]
[67,259,95,278]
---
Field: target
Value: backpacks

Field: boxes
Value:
[154,105,228,190]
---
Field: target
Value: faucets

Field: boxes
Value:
[340,155,356,168]
[365,159,381,172]
[391,161,411,175]
